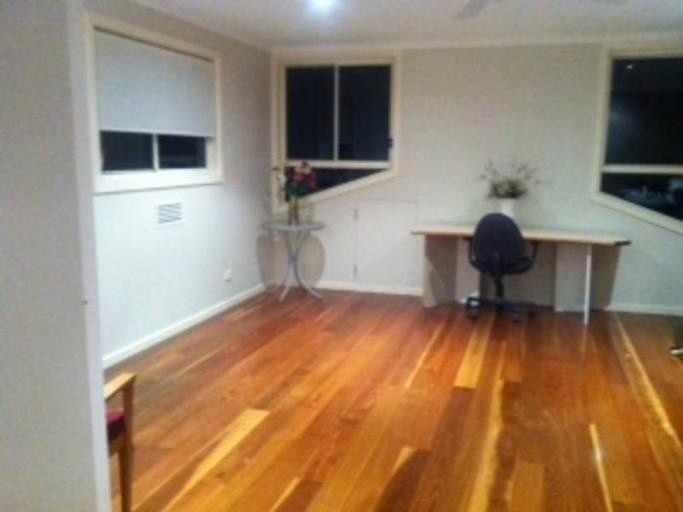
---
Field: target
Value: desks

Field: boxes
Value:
[263,220,326,302]
[409,223,630,329]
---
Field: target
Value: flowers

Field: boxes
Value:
[281,160,318,221]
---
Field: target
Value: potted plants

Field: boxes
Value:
[483,158,542,221]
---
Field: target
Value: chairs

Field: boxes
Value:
[464,213,536,323]
